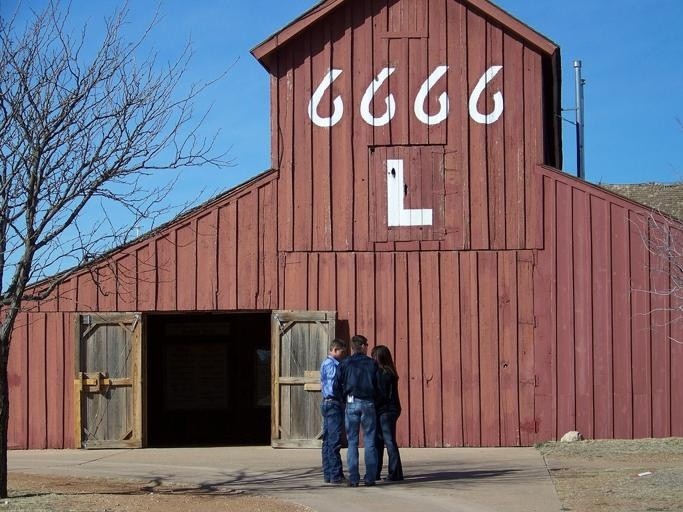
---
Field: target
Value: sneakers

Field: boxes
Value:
[383,475,405,483]
[324,474,358,487]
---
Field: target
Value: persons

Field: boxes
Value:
[336,335,386,487]
[321,339,350,486]
[364,345,403,481]
